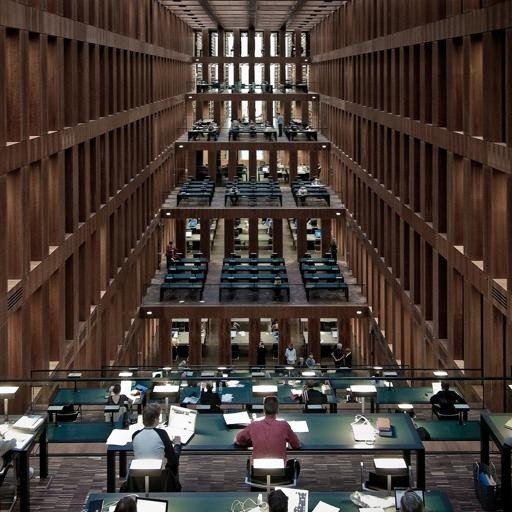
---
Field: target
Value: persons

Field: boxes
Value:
[276,113,283,137]
[166,241,174,269]
[299,383,315,410]
[229,184,239,206]
[107,384,130,426]
[236,397,301,492]
[198,382,224,413]
[331,343,352,373]
[114,497,137,512]
[132,404,181,477]
[331,241,338,264]
[430,382,467,415]
[298,186,307,206]
[267,489,288,512]
[399,490,423,512]
[285,344,321,369]
[188,218,197,224]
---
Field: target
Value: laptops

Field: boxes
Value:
[199,381,216,399]
[165,405,199,444]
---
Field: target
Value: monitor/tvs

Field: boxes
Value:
[432,382,443,394]
[273,486,308,512]
[136,498,168,512]
[394,486,425,510]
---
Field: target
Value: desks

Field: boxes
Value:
[0,373,512,512]
[160,180,348,301]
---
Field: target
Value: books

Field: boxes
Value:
[4,416,45,451]
[376,417,393,437]
